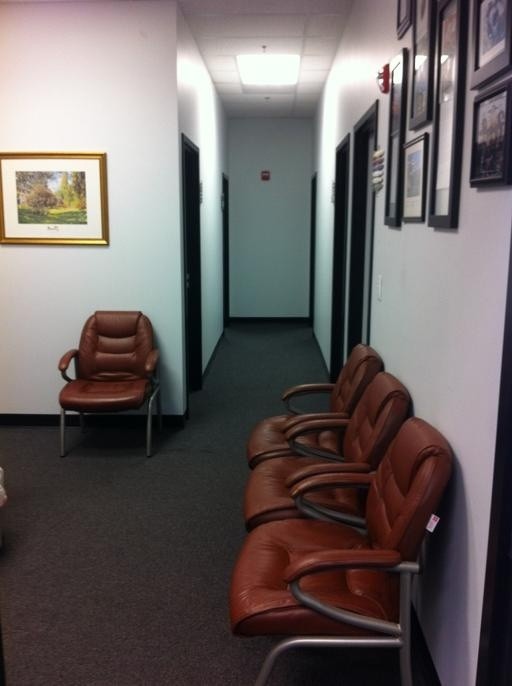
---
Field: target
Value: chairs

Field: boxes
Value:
[58,309,164,459]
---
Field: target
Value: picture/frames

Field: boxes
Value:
[0,151,112,248]
[384,0,511,230]
